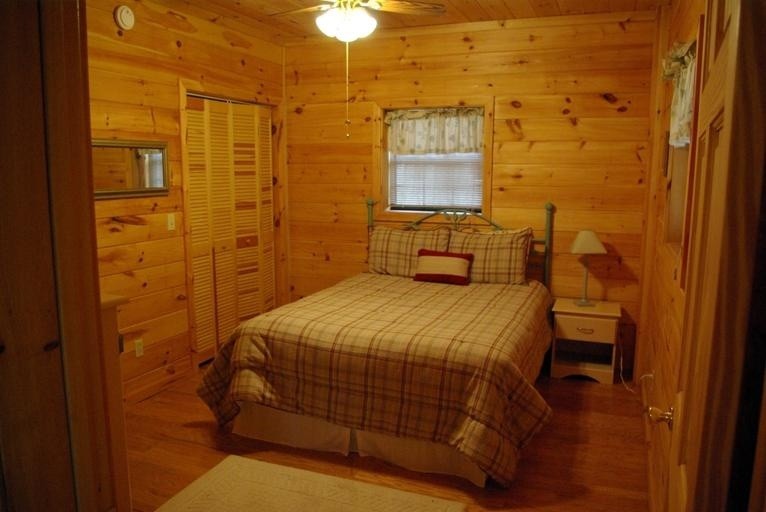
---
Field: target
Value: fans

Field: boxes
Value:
[260,1,447,24]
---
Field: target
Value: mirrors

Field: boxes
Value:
[91,141,169,195]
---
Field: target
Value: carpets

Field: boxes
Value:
[144,450,466,512]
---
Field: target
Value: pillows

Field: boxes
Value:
[411,247,475,288]
[365,225,449,279]
[449,226,533,288]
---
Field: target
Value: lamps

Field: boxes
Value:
[315,1,376,45]
[568,228,608,308]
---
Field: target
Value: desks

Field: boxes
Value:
[550,296,623,388]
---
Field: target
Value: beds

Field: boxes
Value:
[199,199,556,490]
